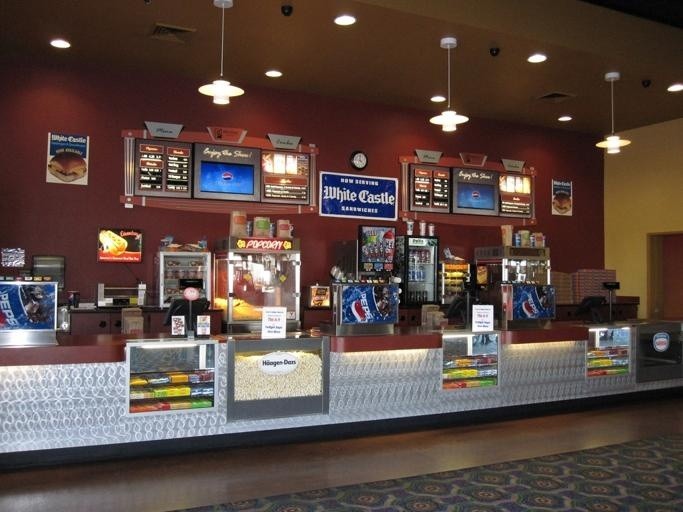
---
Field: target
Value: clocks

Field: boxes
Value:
[349,150,369,170]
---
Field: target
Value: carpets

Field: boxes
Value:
[178,426,682,509]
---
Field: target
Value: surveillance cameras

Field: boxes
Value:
[488,46,501,56]
[280,3,296,16]
[641,79,652,87]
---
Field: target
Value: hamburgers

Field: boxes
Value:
[48,151,87,182]
[552,193,572,214]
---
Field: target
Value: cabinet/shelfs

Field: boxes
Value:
[578,322,630,376]
[409,262,435,304]
[429,328,500,391]
[593,304,609,321]
[439,306,462,325]
[148,313,223,337]
[396,308,422,326]
[63,311,148,338]
[125,339,218,413]
[152,250,212,311]
[608,305,639,321]
[556,306,578,325]
[301,308,335,329]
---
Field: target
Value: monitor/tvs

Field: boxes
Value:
[446,297,481,329]
[164,299,210,335]
[575,296,607,324]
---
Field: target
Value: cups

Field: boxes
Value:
[68,291,81,307]
[405,218,435,237]
[501,225,545,248]
[347,286,388,321]
[167,269,203,280]
[513,286,548,319]
[166,286,179,294]
[0,286,39,328]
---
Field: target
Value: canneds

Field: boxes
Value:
[409,270,426,282]
[409,250,430,263]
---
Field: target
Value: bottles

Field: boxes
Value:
[362,228,395,258]
[410,248,430,304]
[161,239,207,252]
[98,231,128,255]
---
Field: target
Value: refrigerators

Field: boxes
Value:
[396,235,439,308]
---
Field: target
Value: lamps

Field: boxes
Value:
[194,0,246,104]
[593,70,632,156]
[426,35,469,133]
